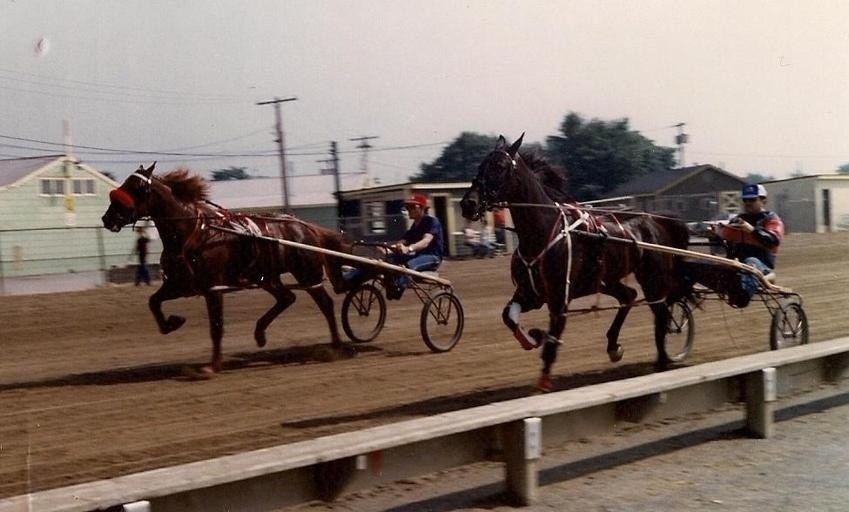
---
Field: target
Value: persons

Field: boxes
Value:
[337,193,445,300]
[462,207,506,259]
[709,184,784,309]
[126,229,155,288]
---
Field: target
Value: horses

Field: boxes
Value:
[101,160,344,374]
[459,130,706,397]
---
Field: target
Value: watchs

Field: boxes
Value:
[408,246,414,253]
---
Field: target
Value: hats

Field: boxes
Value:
[405,194,427,208]
[739,183,767,200]
